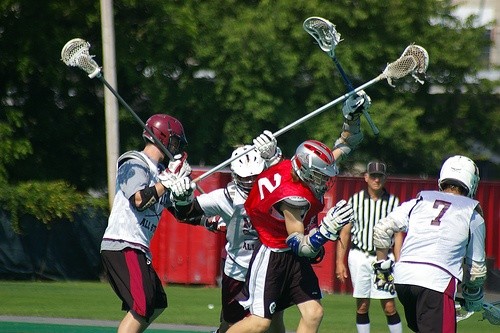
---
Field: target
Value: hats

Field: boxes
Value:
[366,162,386,175]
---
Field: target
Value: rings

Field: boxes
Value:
[337,276,340,278]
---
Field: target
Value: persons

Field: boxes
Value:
[168,130,286,333]
[372,155,487,333]
[100,114,227,333]
[225,89,371,333]
[336,159,406,333]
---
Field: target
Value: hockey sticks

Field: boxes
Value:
[190,44,430,183]
[303,16,380,136]
[61,38,206,194]
[455,297,500,325]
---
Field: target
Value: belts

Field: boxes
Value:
[352,244,390,254]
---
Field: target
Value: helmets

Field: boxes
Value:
[229,145,268,189]
[142,114,187,157]
[437,155,479,198]
[291,140,339,197]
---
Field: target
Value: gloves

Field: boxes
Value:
[158,152,192,190]
[319,199,355,241]
[252,130,282,167]
[342,89,372,120]
[459,284,484,312]
[370,256,396,295]
[170,177,197,206]
